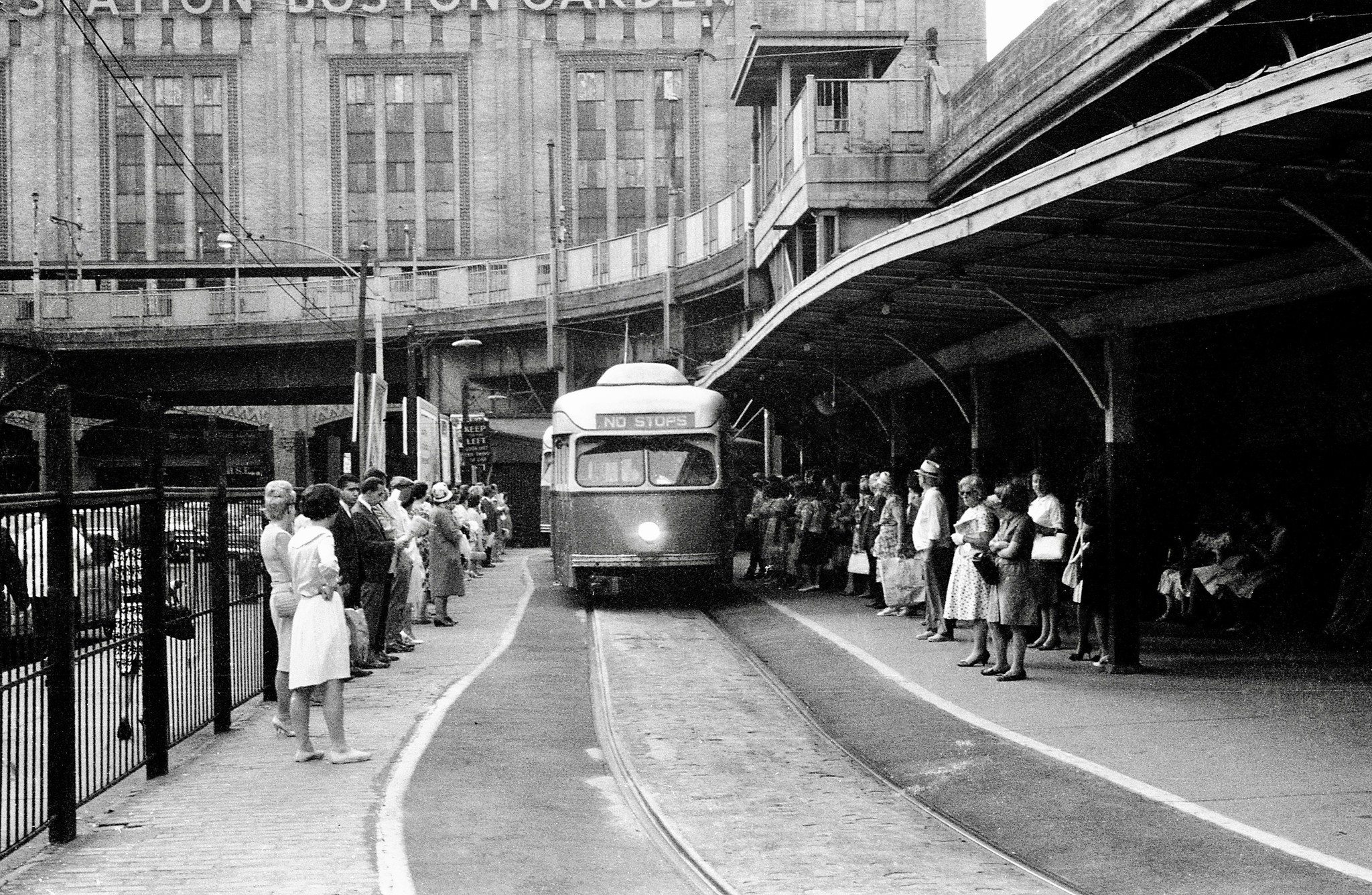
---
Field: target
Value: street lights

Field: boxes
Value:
[215,232,370,481]
[402,322,481,479]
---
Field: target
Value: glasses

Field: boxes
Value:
[961,490,975,496]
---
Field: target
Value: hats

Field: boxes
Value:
[914,460,940,480]
[876,471,895,487]
[390,476,414,489]
[432,482,453,502]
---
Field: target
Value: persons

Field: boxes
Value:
[355,466,437,669]
[1321,492,1372,649]
[1029,466,1109,667]
[914,460,955,641]
[0,527,31,642]
[110,519,183,739]
[943,476,992,666]
[448,481,512,578]
[980,477,1036,681]
[289,484,372,764]
[18,511,92,686]
[1157,505,1291,633]
[429,481,466,627]
[335,474,373,683]
[259,481,323,737]
[737,470,928,625]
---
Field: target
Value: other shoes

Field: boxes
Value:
[338,675,355,683]
[739,566,865,596]
[385,604,457,652]
[350,667,373,678]
[297,749,324,761]
[332,748,370,763]
[367,659,388,669]
[914,620,1138,680]
[360,661,373,669]
[309,694,323,706]
[857,588,956,642]
[374,650,399,662]
[117,719,132,740]
[464,548,507,582]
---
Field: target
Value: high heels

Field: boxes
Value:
[271,717,296,737]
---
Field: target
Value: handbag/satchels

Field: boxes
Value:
[972,552,1004,585]
[162,587,196,640]
[848,549,870,575]
[1061,560,1083,587]
[1031,532,1065,560]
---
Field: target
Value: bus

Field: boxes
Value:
[540,362,739,609]
